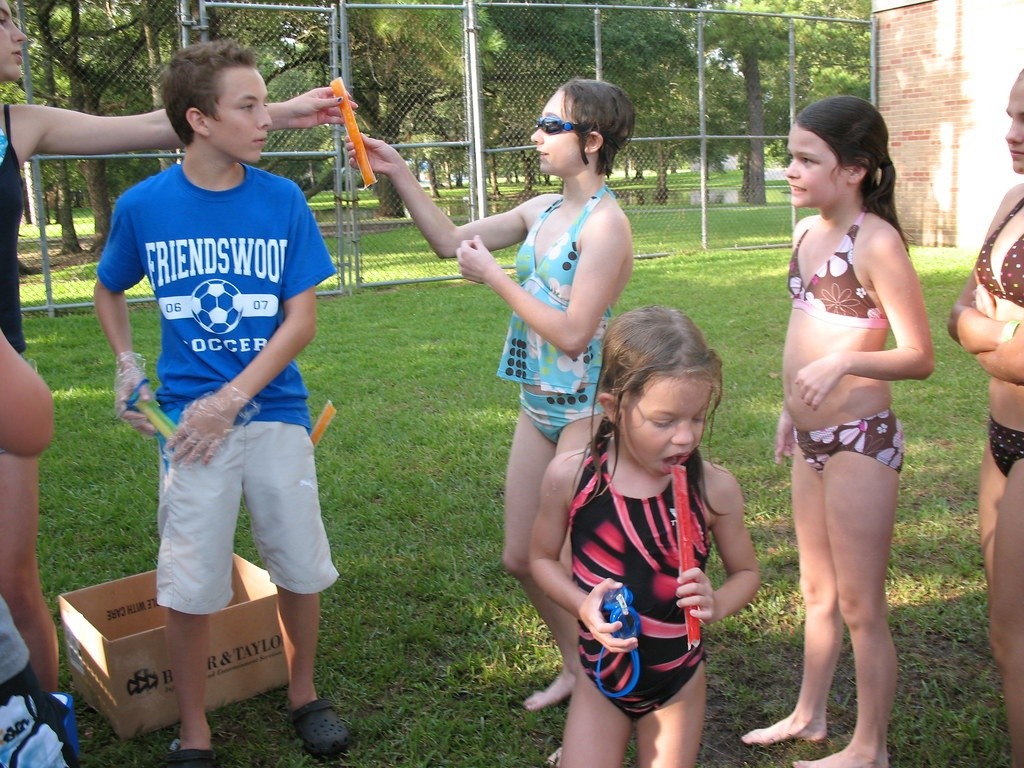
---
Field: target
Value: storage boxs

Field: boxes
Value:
[58,553,289,740]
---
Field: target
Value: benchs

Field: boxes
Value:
[487,201,508,213]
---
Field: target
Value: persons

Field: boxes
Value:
[340,77,635,711]
[526,304,762,768]
[0,333,87,767]
[943,70,1024,768]
[91,39,353,768]
[0,2,358,705]
[742,93,936,767]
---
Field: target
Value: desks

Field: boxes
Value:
[434,199,468,217]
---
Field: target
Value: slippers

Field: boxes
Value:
[166,749,216,768]
[291,698,349,756]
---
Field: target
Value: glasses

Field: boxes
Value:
[596,587,640,697]
[537,116,588,135]
